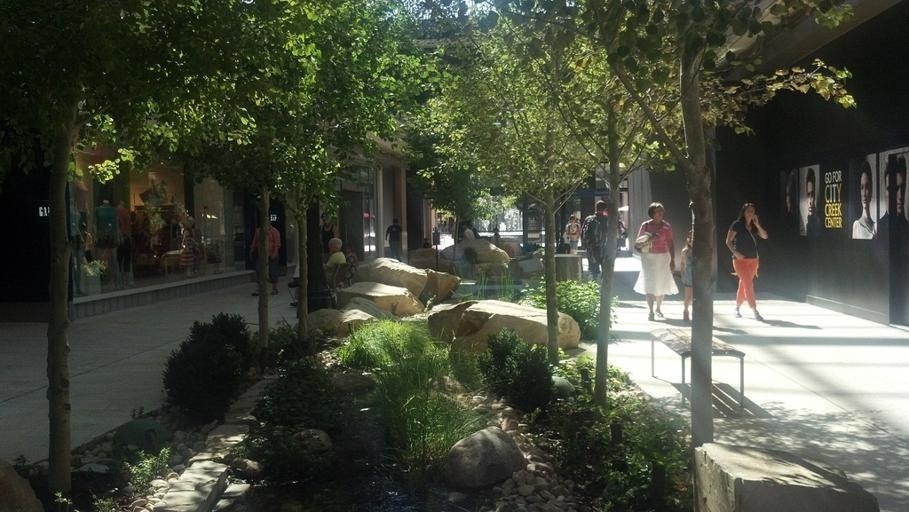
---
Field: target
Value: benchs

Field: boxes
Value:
[649,328,745,408]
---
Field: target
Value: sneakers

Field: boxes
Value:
[252,292,259,296]
[753,311,763,322]
[736,310,741,318]
[271,288,279,295]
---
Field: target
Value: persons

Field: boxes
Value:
[725,202,769,321]
[68,168,223,299]
[679,229,692,321]
[895,158,908,239]
[289,261,299,306]
[492,229,501,244]
[341,240,361,267]
[633,201,679,321]
[251,216,282,296]
[782,172,798,234]
[439,217,480,243]
[806,167,820,236]
[323,237,347,284]
[556,200,628,283]
[320,211,340,264]
[386,218,403,262]
[851,161,876,240]
[879,166,889,247]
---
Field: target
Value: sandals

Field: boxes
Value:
[655,311,663,318]
[648,313,654,320]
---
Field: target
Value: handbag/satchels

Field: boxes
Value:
[637,222,649,253]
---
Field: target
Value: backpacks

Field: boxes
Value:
[582,214,605,249]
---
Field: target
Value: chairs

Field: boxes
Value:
[326,263,353,292]
